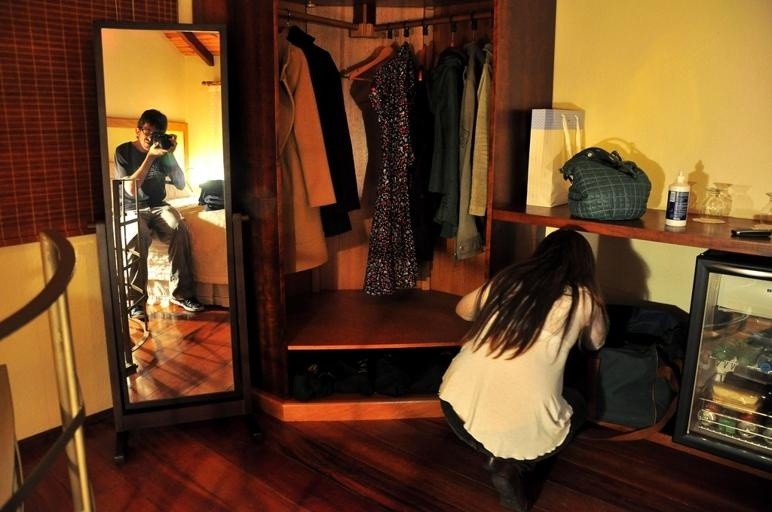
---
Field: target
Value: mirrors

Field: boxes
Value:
[91,18,253,469]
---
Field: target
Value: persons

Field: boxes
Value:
[437,227,611,512]
[113,109,206,321]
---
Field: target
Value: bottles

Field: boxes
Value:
[712,336,772,375]
[665,170,690,228]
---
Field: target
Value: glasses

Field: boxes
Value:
[140,128,154,135]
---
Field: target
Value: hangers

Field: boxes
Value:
[278,6,295,39]
[349,16,495,85]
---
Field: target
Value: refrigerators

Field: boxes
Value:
[672,249,772,474]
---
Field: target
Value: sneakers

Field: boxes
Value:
[169,298,204,312]
[491,459,528,510]
[131,306,144,316]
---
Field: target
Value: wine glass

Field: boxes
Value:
[698,188,728,220]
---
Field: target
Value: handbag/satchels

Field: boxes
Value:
[560,148,651,223]
[525,108,585,217]
[595,301,687,427]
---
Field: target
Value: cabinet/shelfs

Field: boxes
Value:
[493,190,771,485]
[230,0,556,429]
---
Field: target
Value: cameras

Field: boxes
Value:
[151,133,173,148]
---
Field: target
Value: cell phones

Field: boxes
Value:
[731,228,771,237]
